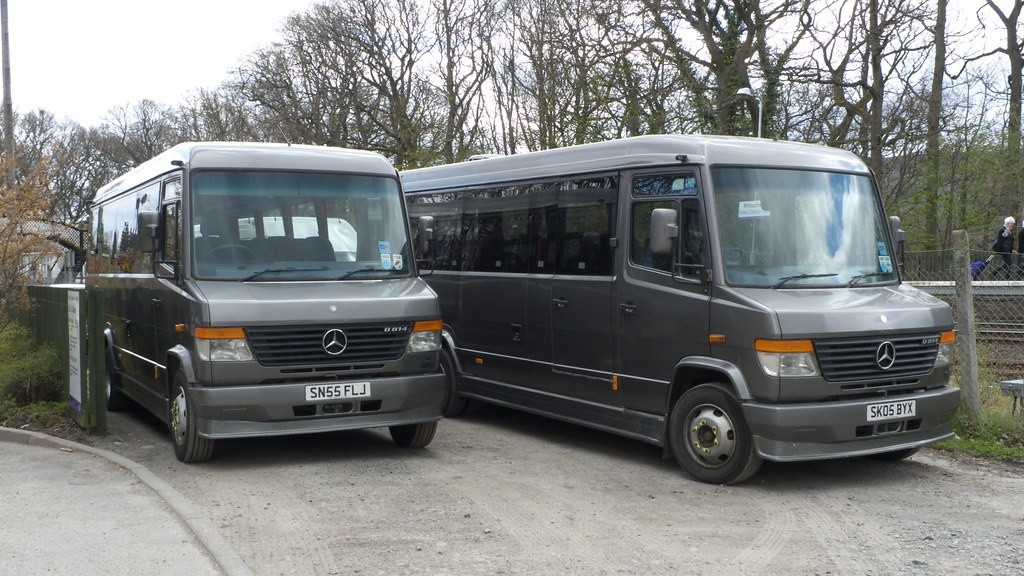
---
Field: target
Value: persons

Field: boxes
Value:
[990,216,1024,281]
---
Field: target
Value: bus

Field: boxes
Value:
[368,135,961,484]
[85,140,448,463]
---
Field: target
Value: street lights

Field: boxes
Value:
[737,87,763,265]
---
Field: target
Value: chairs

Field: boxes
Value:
[462,231,610,274]
[195,210,230,260]
[241,235,334,259]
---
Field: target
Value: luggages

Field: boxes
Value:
[971,254,995,281]
